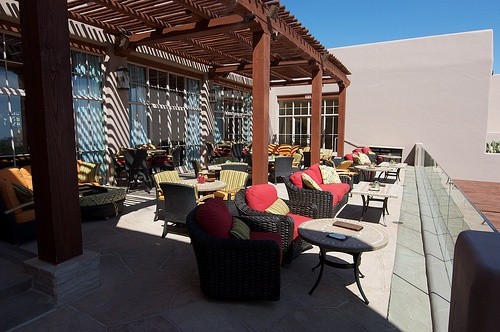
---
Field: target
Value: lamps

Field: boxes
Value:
[116,66,130,89]
[208,89,218,103]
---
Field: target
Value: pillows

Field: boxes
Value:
[302,173,323,191]
[319,165,342,185]
[359,153,371,164]
[11,183,34,209]
[266,198,290,216]
[230,216,251,240]
[353,154,363,165]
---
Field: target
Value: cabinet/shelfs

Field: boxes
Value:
[370,146,405,164]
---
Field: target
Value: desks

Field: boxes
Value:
[178,179,225,195]
[353,165,389,183]
[349,180,400,227]
[296,217,390,304]
[378,161,408,183]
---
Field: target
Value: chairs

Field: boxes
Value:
[0,140,379,304]
